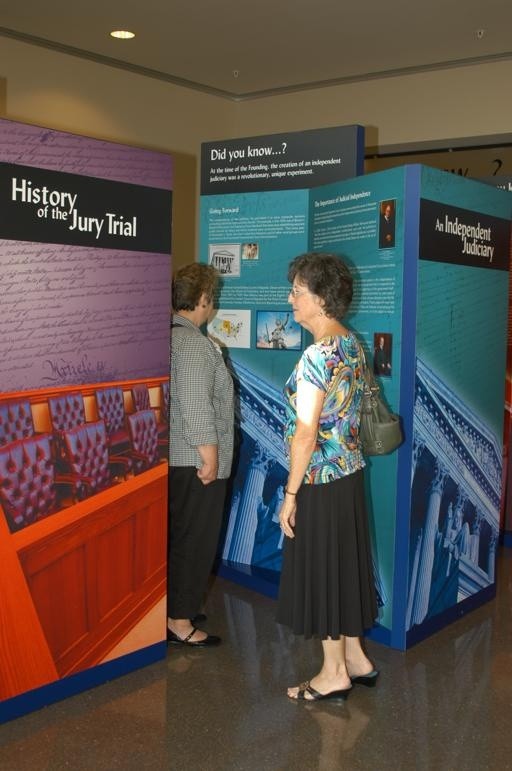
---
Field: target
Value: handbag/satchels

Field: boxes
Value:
[359,385,403,458]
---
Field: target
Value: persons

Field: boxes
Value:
[245,244,251,258]
[373,334,392,376]
[250,244,258,258]
[447,508,464,545]
[379,202,394,248]
[264,310,290,348]
[165,256,244,653]
[286,687,378,771]
[273,249,383,708]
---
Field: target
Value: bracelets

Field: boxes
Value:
[284,490,298,496]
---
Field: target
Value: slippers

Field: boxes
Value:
[346,671,382,690]
[288,680,353,704]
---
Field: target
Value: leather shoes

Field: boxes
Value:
[192,614,208,625]
[167,625,222,647]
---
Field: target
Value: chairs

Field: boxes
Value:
[1,381,170,537]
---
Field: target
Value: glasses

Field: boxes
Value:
[285,287,307,300]
